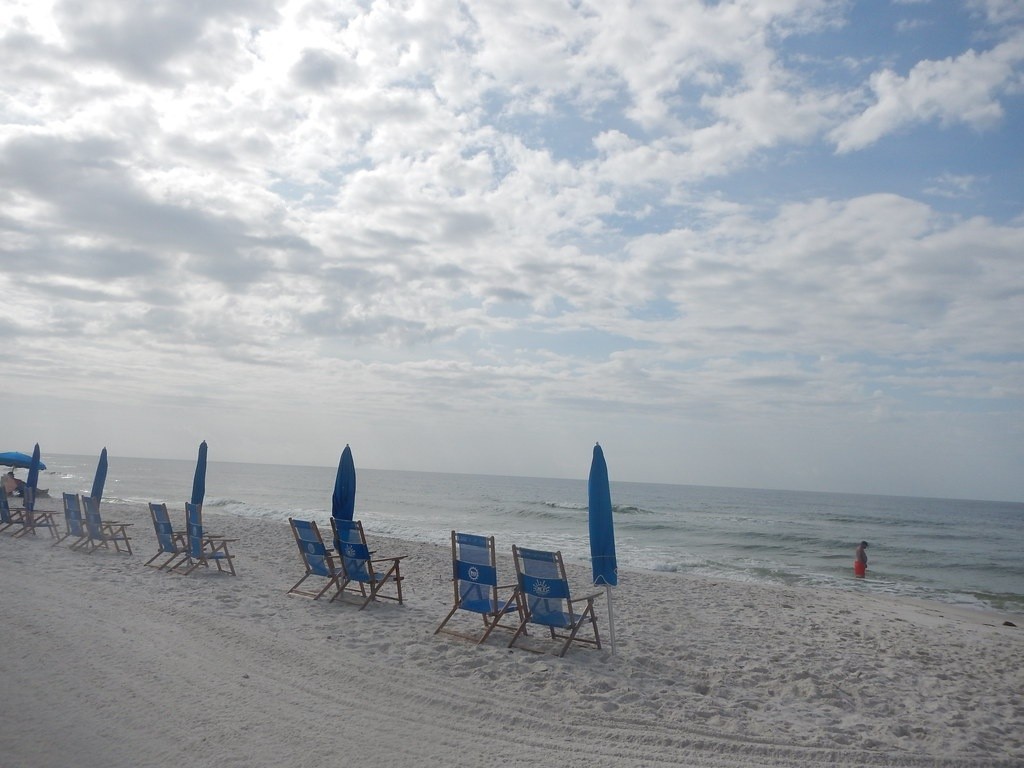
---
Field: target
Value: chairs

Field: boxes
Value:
[287,518,409,612]
[0,491,135,556]
[433,529,603,660]
[144,502,241,576]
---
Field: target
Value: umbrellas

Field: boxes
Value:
[23,443,40,508]
[0,451,46,472]
[332,444,356,550]
[191,440,207,504]
[90,446,108,500]
[588,442,617,655]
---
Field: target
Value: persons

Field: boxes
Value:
[854,541,868,577]
[8,472,49,493]
